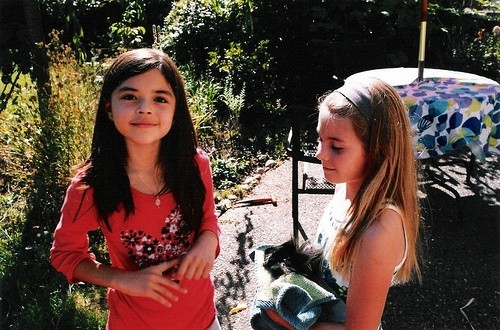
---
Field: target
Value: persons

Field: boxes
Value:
[249,73,421,330]
[49,47,223,330]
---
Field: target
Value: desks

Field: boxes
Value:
[344,67,500,186]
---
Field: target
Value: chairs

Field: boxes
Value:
[287,106,334,236]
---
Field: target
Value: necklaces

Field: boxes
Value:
[127,163,165,207]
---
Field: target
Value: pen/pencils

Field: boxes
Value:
[237,198,278,207]
